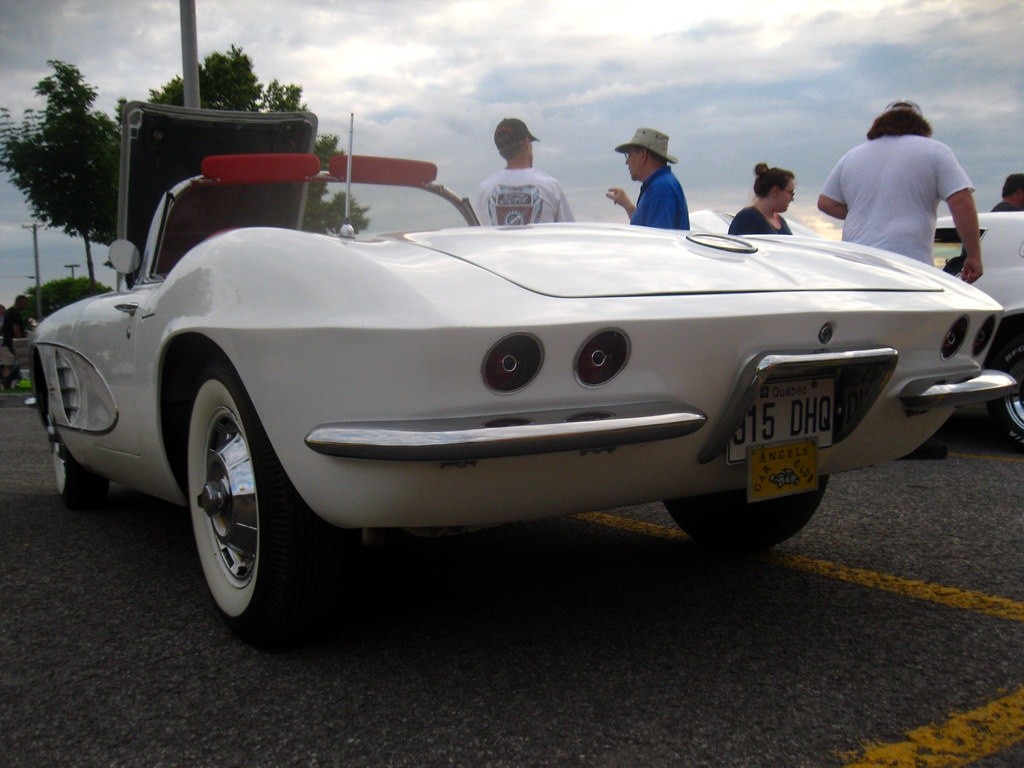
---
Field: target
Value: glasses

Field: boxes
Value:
[779,188,795,198]
[625,148,649,161]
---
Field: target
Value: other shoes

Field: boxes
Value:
[896,434,948,462]
[3,379,11,390]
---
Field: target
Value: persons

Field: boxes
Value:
[728,163,796,235]
[818,100,983,284]
[991,174,1024,212]
[606,128,690,230]
[0,295,37,387]
[475,118,576,226]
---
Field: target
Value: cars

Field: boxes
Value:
[931,212,1024,451]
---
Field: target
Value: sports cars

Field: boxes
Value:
[26,103,1019,642]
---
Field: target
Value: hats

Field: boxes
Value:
[615,128,678,163]
[883,100,922,118]
[494,118,540,149]
